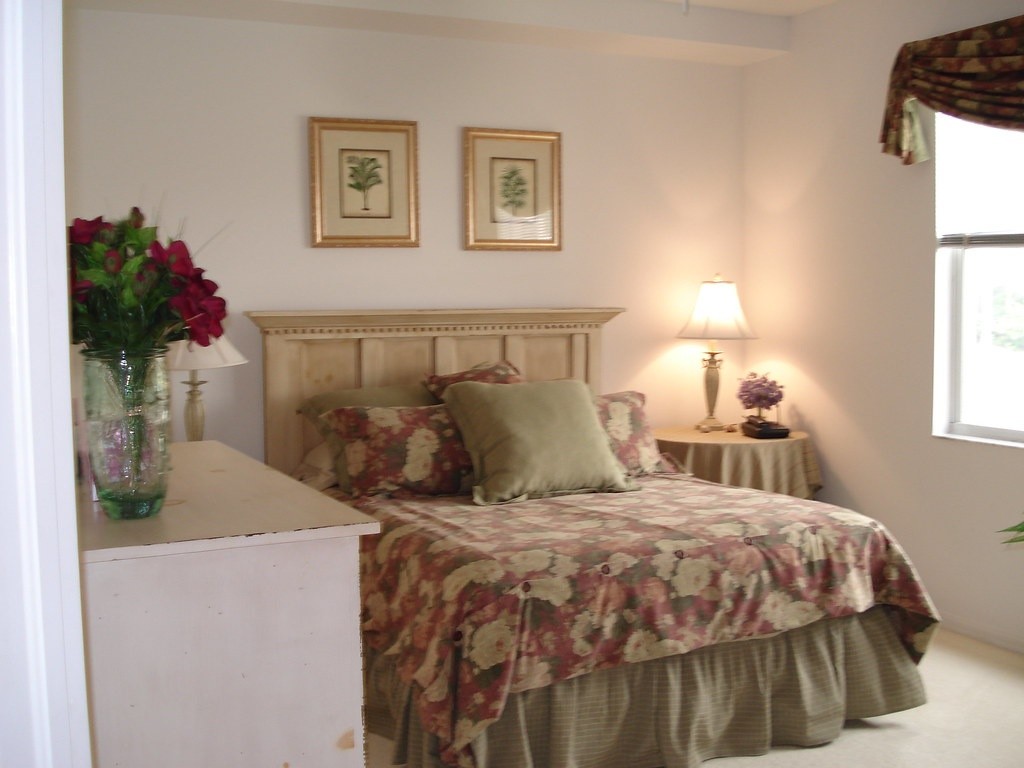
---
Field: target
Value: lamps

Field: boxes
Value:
[676,281,758,432]
[166,332,249,443]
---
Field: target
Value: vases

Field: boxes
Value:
[78,351,170,519]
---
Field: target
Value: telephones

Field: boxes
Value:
[743,415,791,441]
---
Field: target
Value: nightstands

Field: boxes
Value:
[651,426,824,500]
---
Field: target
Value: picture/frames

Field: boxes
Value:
[308,117,419,246]
[461,127,562,248]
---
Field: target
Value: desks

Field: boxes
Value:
[75,439,383,768]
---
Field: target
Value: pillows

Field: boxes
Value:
[295,384,434,495]
[590,390,674,474]
[441,380,643,507]
[421,360,525,408]
[319,403,475,498]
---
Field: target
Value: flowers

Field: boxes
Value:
[736,372,784,416]
[67,207,226,350]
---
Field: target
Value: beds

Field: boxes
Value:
[245,305,941,768]
